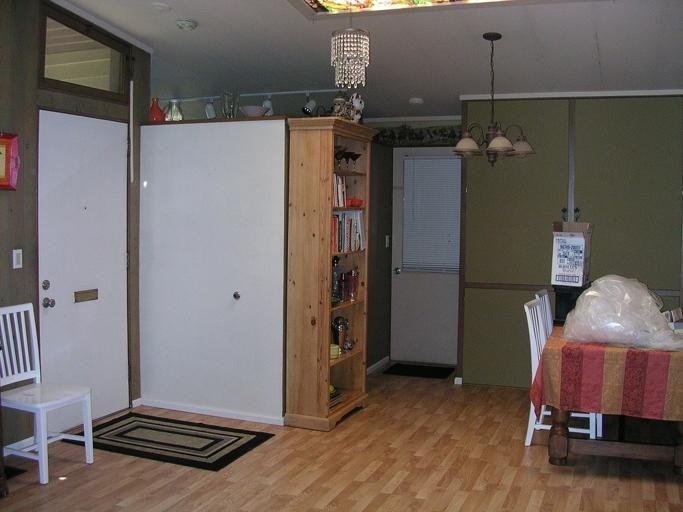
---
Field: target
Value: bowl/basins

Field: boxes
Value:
[238,107,269,117]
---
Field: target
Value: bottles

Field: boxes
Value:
[330,257,360,305]
[146,97,184,121]
[332,316,354,352]
[203,95,217,120]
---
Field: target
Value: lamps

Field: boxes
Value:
[450,33,535,167]
[328,1,372,91]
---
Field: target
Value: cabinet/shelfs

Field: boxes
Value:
[286,116,377,434]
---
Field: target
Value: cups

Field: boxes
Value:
[219,87,237,119]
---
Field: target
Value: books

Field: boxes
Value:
[330,169,367,256]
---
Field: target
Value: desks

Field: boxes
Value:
[538,323,682,469]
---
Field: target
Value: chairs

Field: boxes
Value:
[1,302,93,488]
[522,287,607,448]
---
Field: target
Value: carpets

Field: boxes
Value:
[61,411,274,474]
